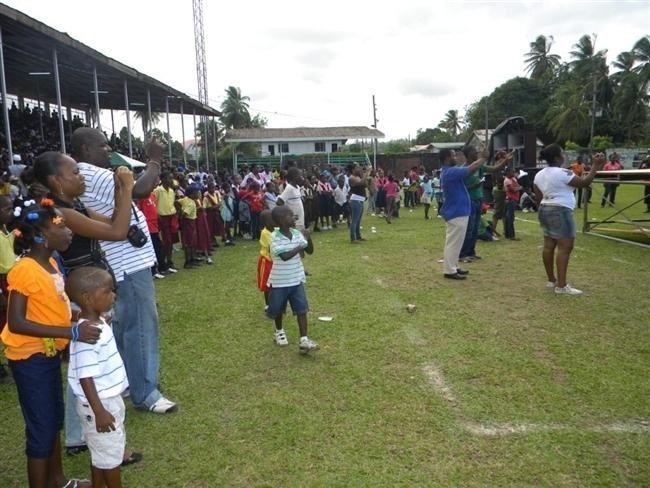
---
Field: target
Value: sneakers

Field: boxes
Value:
[272,329,288,345]
[546,281,560,287]
[300,338,319,353]
[149,398,178,414]
[555,284,584,297]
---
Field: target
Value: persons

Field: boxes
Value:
[348,161,372,242]
[638,148,650,213]
[460,146,514,262]
[383,176,400,224]
[191,188,212,265]
[503,165,523,241]
[64,265,129,487]
[376,169,385,214]
[419,176,434,219]
[179,184,201,268]
[565,152,595,209]
[368,170,376,214]
[437,148,490,280]
[0,200,92,488]
[519,187,537,212]
[258,211,275,311]
[258,150,282,205]
[410,167,418,206]
[136,192,165,280]
[231,162,262,240]
[533,144,605,296]
[221,183,234,240]
[302,162,349,231]
[267,206,321,356]
[402,170,410,207]
[275,168,305,232]
[203,184,234,247]
[492,178,507,236]
[433,170,443,218]
[0,100,147,177]
[20,152,134,299]
[71,128,180,414]
[476,204,502,241]
[9,154,26,177]
[602,154,622,207]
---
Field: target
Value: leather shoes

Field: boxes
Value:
[444,272,466,279]
[457,268,468,273]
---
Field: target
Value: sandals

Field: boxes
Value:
[60,478,93,487]
